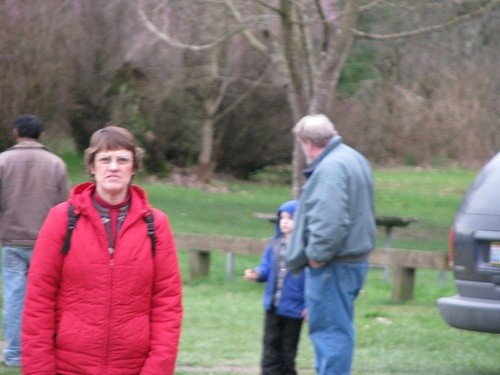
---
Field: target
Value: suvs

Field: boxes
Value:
[436,151,500,333]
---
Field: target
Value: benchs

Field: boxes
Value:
[255,212,420,248]
[174,232,449,303]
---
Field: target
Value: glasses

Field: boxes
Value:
[93,155,136,169]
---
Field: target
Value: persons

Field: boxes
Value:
[20,126,183,375]
[243,200,308,375]
[0,115,71,367]
[285,113,378,375]
